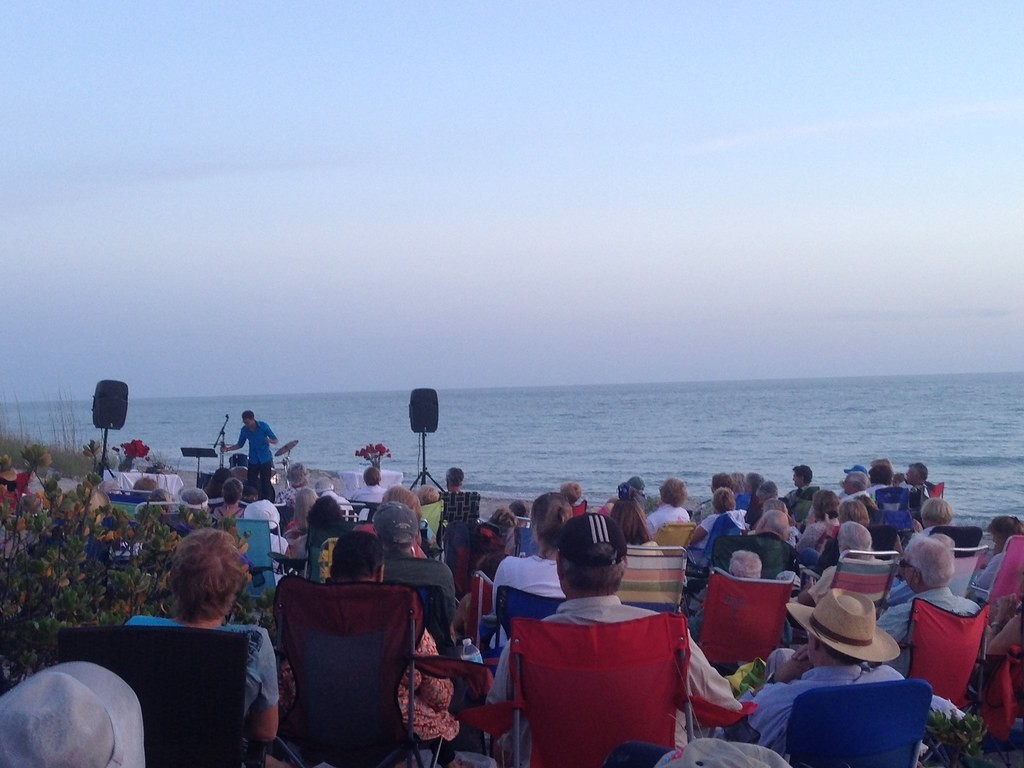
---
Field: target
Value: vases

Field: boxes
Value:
[118,456,134,472]
[370,460,382,472]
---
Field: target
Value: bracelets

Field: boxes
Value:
[767,672,776,683]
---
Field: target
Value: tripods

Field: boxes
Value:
[410,433,445,491]
[95,429,126,495]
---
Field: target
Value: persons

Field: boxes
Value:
[0,530,455,768]
[510,499,530,517]
[418,484,439,506]
[99,477,159,498]
[180,461,423,575]
[723,584,965,768]
[446,467,464,493]
[220,410,279,501]
[597,476,687,555]
[560,482,582,504]
[371,491,743,768]
[968,515,1024,705]
[688,457,954,671]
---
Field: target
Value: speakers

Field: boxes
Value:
[408,388,439,433]
[91,380,128,430]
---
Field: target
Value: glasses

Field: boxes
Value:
[899,559,921,582]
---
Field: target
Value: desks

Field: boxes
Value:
[336,469,404,494]
[102,470,183,494]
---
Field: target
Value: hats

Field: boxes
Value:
[550,513,626,566]
[844,465,867,475]
[0,661,145,768]
[786,588,901,661]
[244,499,281,529]
[373,500,418,545]
[628,477,644,489]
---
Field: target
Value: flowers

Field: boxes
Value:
[112,439,150,470]
[355,443,392,468]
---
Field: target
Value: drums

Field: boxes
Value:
[229,453,249,474]
[259,459,276,479]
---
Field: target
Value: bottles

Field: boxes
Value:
[461,638,484,665]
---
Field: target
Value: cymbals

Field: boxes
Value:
[208,442,232,446]
[275,459,295,464]
[274,439,299,457]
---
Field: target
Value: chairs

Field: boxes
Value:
[29,483,1024,768]
